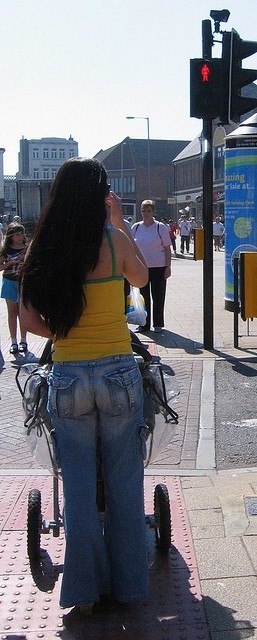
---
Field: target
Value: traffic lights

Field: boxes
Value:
[190,58,224,118]
[217,27,257,128]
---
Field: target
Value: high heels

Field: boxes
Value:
[77,597,100,616]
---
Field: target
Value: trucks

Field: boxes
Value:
[14,178,54,242]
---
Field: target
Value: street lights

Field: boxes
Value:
[120,142,126,198]
[126,116,151,200]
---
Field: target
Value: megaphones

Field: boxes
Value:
[210,7,230,23]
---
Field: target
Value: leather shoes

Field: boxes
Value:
[19,342,27,351]
[10,344,18,353]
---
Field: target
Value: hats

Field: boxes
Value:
[13,215,21,222]
[2,214,9,222]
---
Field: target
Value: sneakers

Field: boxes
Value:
[154,327,161,333]
[134,326,144,332]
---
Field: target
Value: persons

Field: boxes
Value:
[190,217,197,240]
[161,217,171,232]
[168,220,177,255]
[127,218,133,223]
[0,225,30,352]
[0,214,3,243]
[213,217,225,251]
[198,218,202,228]
[1,215,9,232]
[13,216,20,223]
[131,200,171,332]
[177,215,193,253]
[21,158,150,615]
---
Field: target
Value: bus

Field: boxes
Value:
[117,197,136,226]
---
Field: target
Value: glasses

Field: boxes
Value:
[141,209,152,212]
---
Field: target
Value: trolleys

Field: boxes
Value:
[14,328,180,558]
